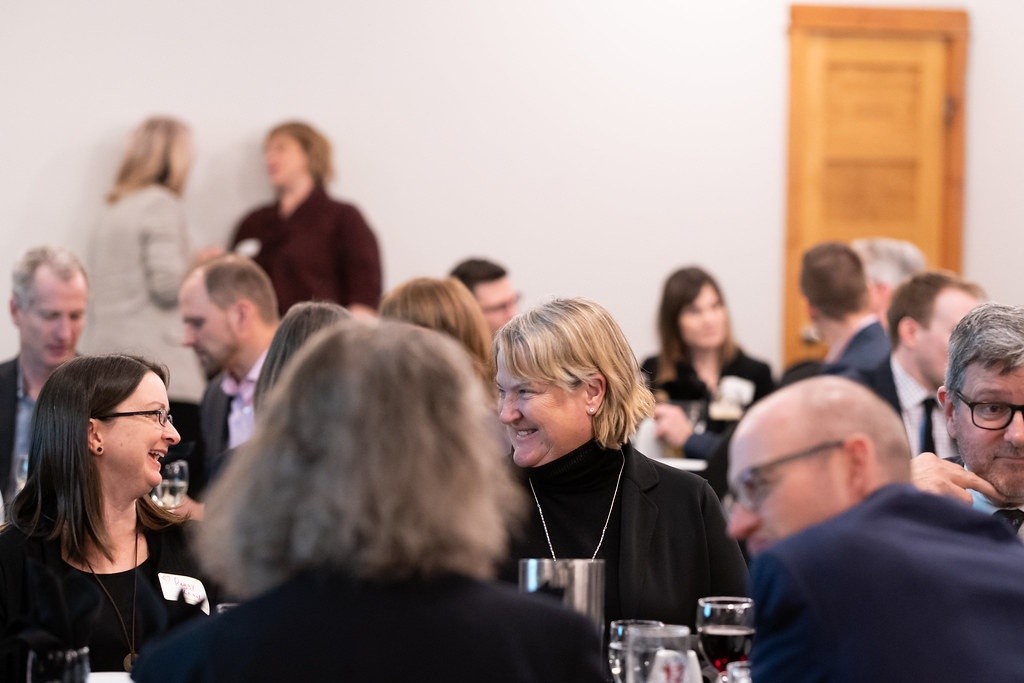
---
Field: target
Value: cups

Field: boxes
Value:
[26,645,91,683]
[152,459,189,513]
[609,619,690,683]
[695,594,756,683]
[16,454,28,489]
[516,559,606,644]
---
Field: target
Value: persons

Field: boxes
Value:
[730,377,1024,683]
[491,298,748,633]
[654,246,901,463]
[642,267,773,432]
[380,259,518,372]
[81,118,225,370]
[0,246,88,516]
[856,238,990,460]
[230,122,381,321]
[130,323,607,683]
[0,355,231,675]
[168,257,350,521]
[910,304,1024,543]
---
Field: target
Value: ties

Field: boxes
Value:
[917,397,940,457]
[990,508,1024,536]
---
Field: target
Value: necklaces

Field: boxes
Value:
[529,448,625,561]
[85,523,139,671]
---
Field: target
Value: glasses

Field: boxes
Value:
[720,439,844,523]
[955,389,1024,430]
[91,408,174,428]
